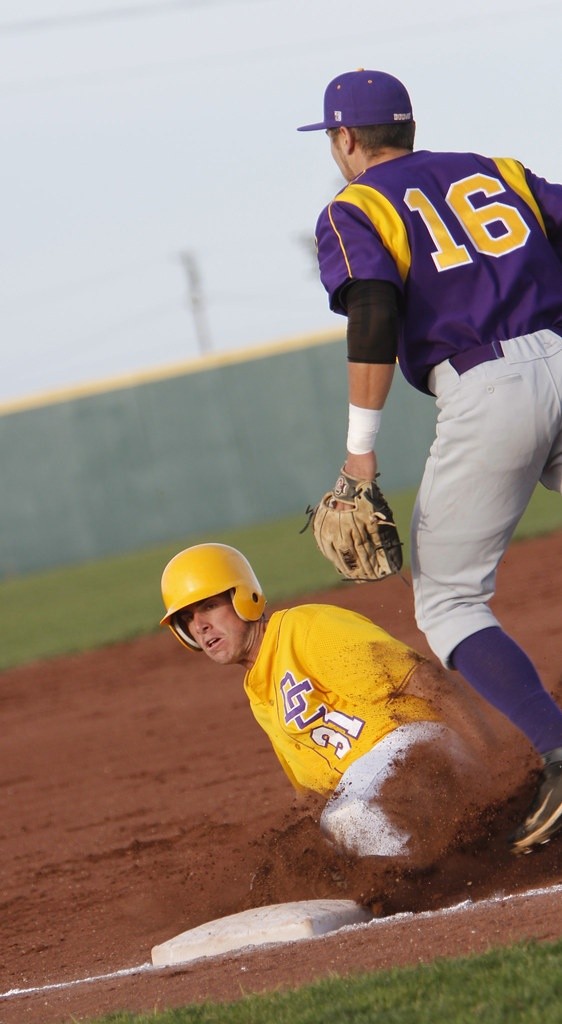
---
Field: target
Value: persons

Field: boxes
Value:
[159,542,478,859]
[298,71,562,852]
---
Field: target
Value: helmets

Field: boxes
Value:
[160,542,269,653]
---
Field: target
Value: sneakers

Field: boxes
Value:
[509,763,562,856]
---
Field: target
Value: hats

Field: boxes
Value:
[296,68,413,131]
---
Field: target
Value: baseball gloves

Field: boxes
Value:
[312,465,406,582]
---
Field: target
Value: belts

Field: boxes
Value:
[448,321,562,376]
